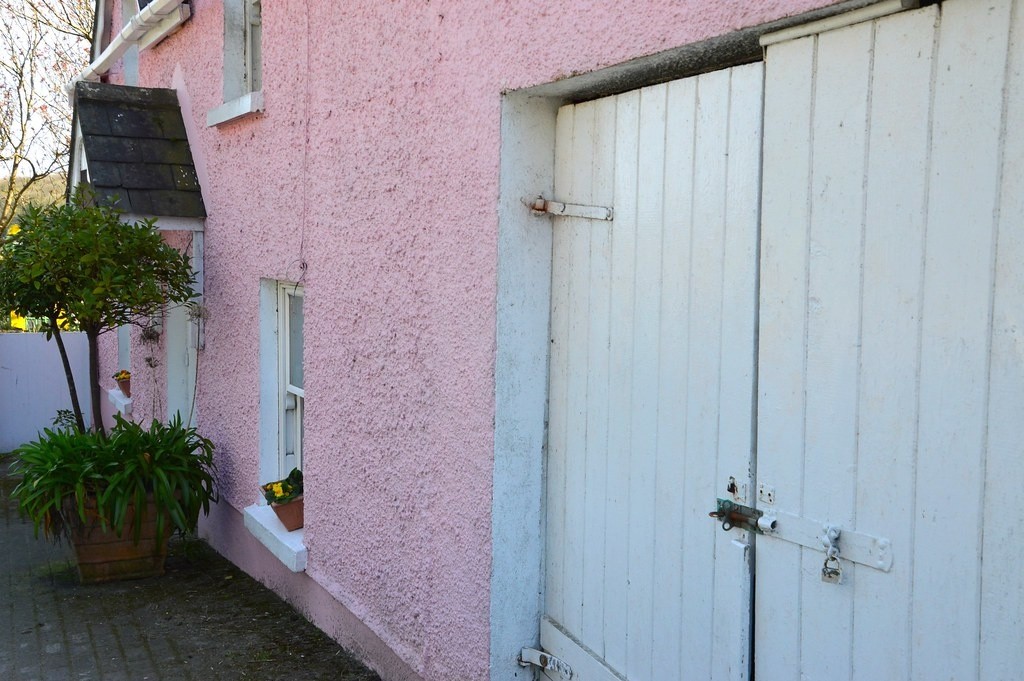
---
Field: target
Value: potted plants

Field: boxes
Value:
[0,177,218,589]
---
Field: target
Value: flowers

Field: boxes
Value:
[262,469,306,508]
[111,369,131,380]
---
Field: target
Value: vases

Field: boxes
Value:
[260,484,303,533]
[116,378,130,397]
